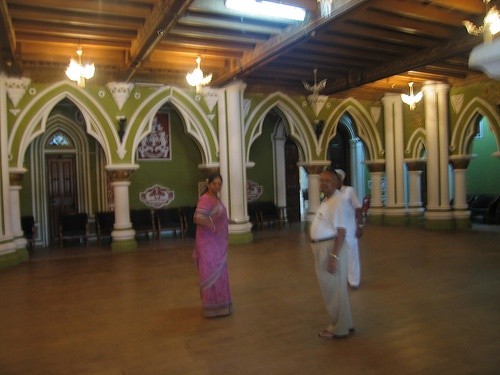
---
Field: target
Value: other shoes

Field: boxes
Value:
[349,327,355,333]
[318,329,347,339]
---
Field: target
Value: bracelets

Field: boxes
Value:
[329,252,341,262]
[358,224,365,229]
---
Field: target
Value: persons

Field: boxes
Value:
[335,170,365,289]
[190,171,234,320]
[308,169,357,340]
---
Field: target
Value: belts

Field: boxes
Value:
[311,238,333,243]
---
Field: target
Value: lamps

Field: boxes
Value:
[301,69,327,97]
[400,81,423,111]
[463,0,500,44]
[64,47,95,88]
[224,0,311,22]
[186,56,212,93]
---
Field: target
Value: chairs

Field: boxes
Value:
[248,201,282,232]
[58,212,89,249]
[94,206,197,241]
[450,193,500,224]
[355,195,370,227]
[20,215,37,246]
